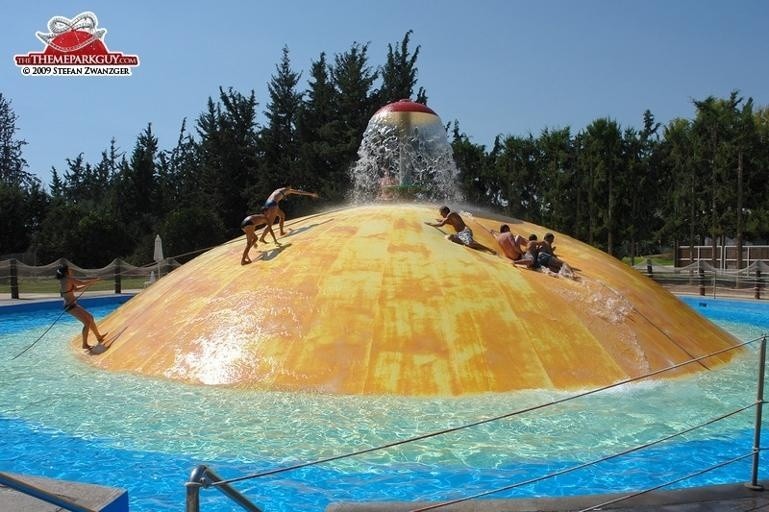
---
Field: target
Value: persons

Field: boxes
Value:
[56,264,108,349]
[424,206,497,255]
[241,184,319,265]
[497,224,580,281]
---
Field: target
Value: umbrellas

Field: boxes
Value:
[154,234,163,279]
